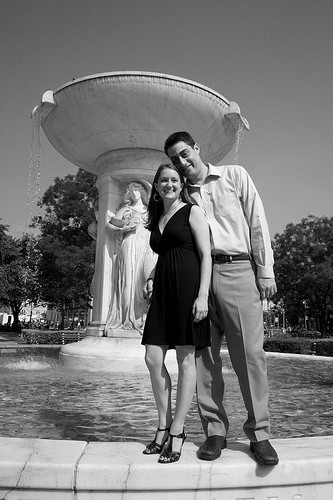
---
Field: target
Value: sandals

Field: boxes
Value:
[142,420,173,454]
[158,428,187,463]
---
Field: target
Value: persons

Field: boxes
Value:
[142,131,280,466]
[104,182,160,337]
[141,164,213,464]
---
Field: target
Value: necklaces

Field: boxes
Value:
[162,201,182,224]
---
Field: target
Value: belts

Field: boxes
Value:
[212,253,251,263]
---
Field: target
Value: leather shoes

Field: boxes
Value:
[197,435,227,461]
[250,440,279,465]
